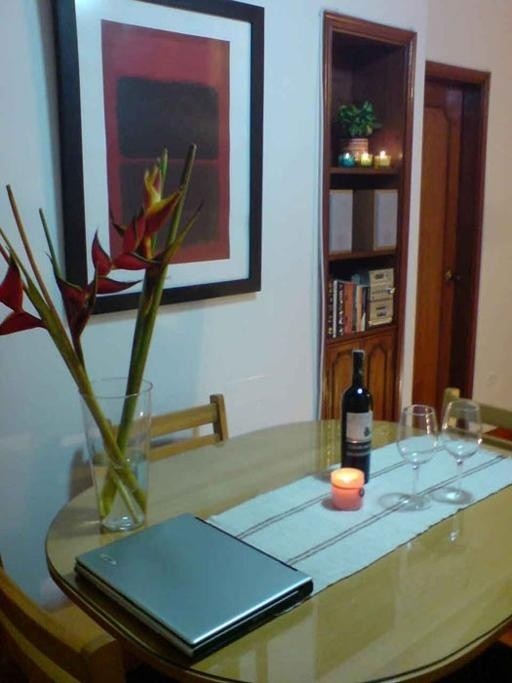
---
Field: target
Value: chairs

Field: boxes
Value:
[0,569,167,683]
[440,388,512,453]
[105,394,230,463]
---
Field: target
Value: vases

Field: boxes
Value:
[78,377,154,531]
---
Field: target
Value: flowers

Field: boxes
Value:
[0,144,198,522]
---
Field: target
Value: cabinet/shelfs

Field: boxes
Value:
[323,10,399,420]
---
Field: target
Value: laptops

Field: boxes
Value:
[73,513,314,660]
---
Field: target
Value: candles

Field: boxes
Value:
[339,152,355,168]
[360,151,373,166]
[374,150,391,167]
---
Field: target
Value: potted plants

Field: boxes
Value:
[338,101,381,161]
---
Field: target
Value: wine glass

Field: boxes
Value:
[397,403,439,511]
[441,399,484,505]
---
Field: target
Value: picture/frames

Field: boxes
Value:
[54,0,264,316]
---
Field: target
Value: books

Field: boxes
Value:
[328,273,368,339]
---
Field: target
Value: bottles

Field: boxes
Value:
[340,348,374,486]
